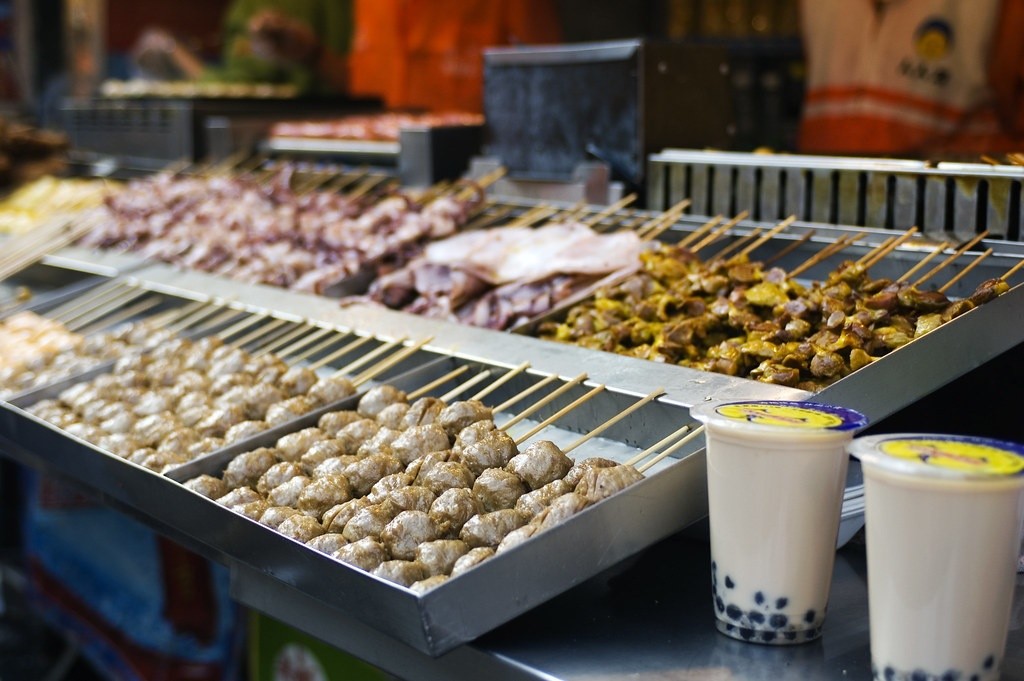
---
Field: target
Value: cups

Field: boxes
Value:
[690,400,868,645]
[849,433,1024,681]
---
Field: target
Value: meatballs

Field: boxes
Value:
[0,319,649,596]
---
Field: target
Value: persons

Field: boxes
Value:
[796,0,1024,164]
[40,0,561,133]
